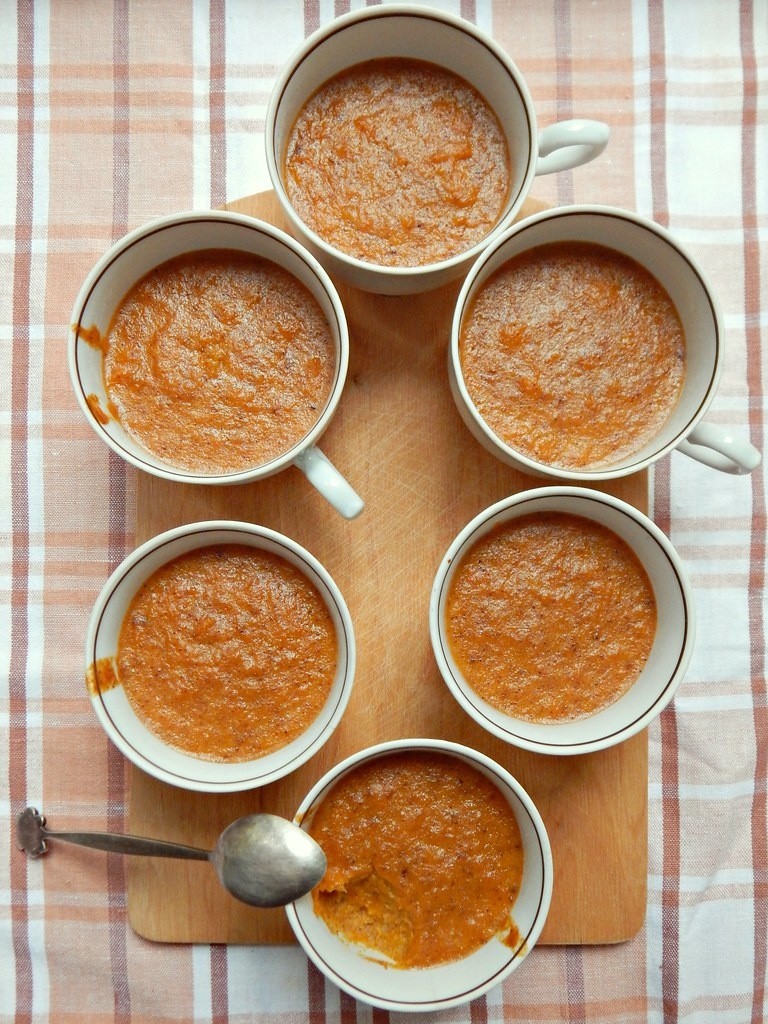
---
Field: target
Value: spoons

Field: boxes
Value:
[15,807,327,909]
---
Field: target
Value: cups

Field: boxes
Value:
[264,3,611,294]
[84,521,356,794]
[428,486,696,756]
[284,738,553,1013]
[67,210,364,520]
[447,203,761,481]
